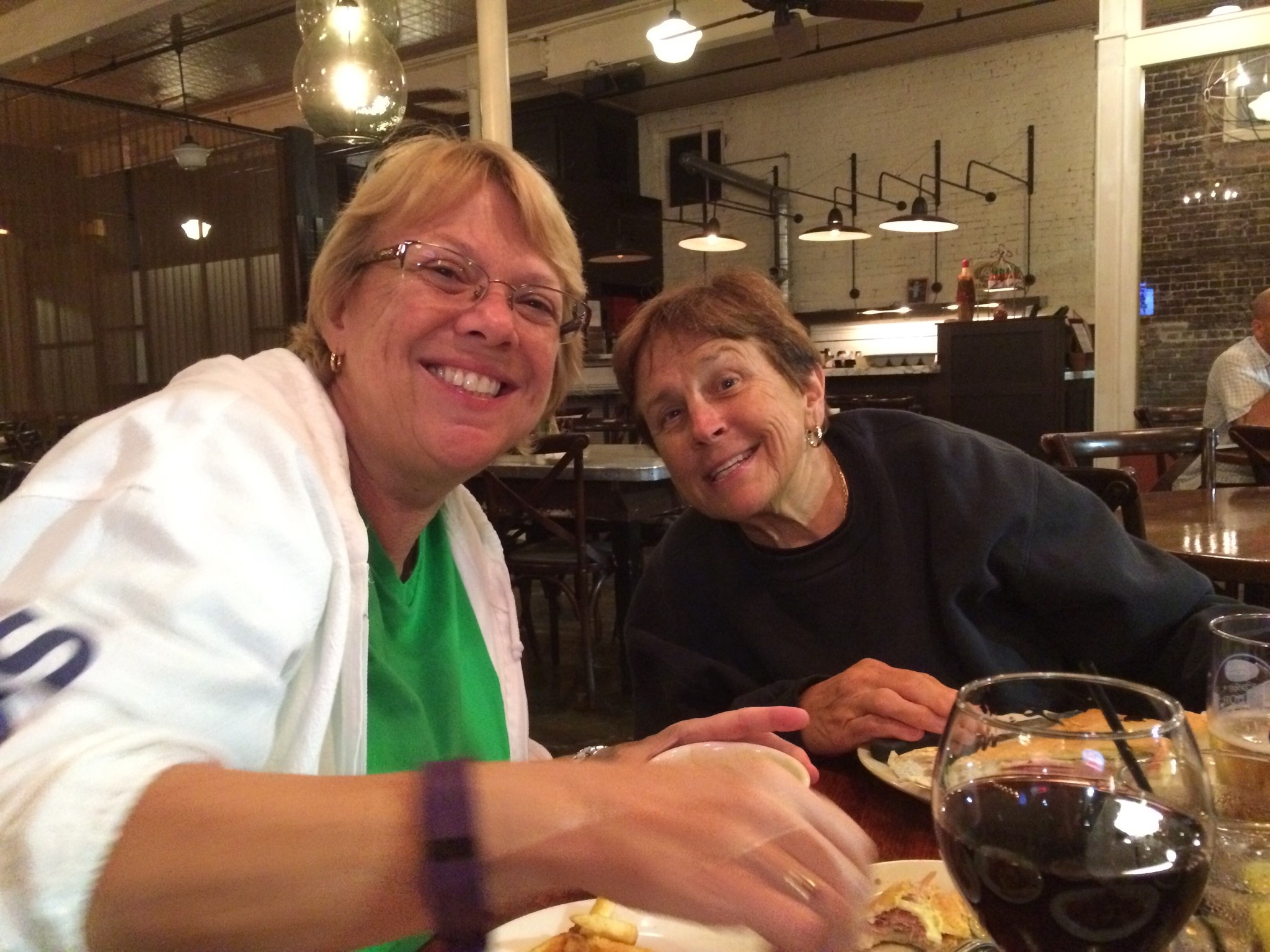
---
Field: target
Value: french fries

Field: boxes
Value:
[530,896,652,952]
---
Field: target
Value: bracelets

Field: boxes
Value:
[416,759,484,946]
[575,745,608,760]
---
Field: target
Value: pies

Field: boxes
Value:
[867,880,972,952]
[888,709,1209,788]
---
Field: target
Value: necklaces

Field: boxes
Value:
[833,456,849,520]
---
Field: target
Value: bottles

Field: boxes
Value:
[958,260,976,321]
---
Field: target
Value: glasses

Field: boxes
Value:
[355,240,591,345]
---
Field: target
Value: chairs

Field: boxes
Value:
[1040,405,1270,543]
[472,432,634,721]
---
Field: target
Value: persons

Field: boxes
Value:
[0,135,873,952]
[1170,288,1270,490]
[613,270,1270,767]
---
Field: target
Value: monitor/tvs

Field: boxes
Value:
[1137,283,1160,319]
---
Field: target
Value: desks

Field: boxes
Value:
[1112,487,1270,610]
[481,444,672,701]
[567,368,623,444]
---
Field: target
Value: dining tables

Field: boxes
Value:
[812,751,1270,952]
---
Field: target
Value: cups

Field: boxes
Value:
[1119,746,1270,952]
[932,671,1214,952]
[1205,612,1270,786]
[645,740,811,790]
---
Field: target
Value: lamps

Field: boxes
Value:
[646,0,703,63]
[170,13,214,172]
[180,210,211,240]
[879,174,996,232]
[587,217,708,263]
[798,188,907,241]
[678,198,803,252]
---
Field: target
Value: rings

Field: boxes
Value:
[785,869,815,900]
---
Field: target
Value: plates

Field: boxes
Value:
[486,900,775,952]
[857,707,1053,804]
[867,859,998,952]
